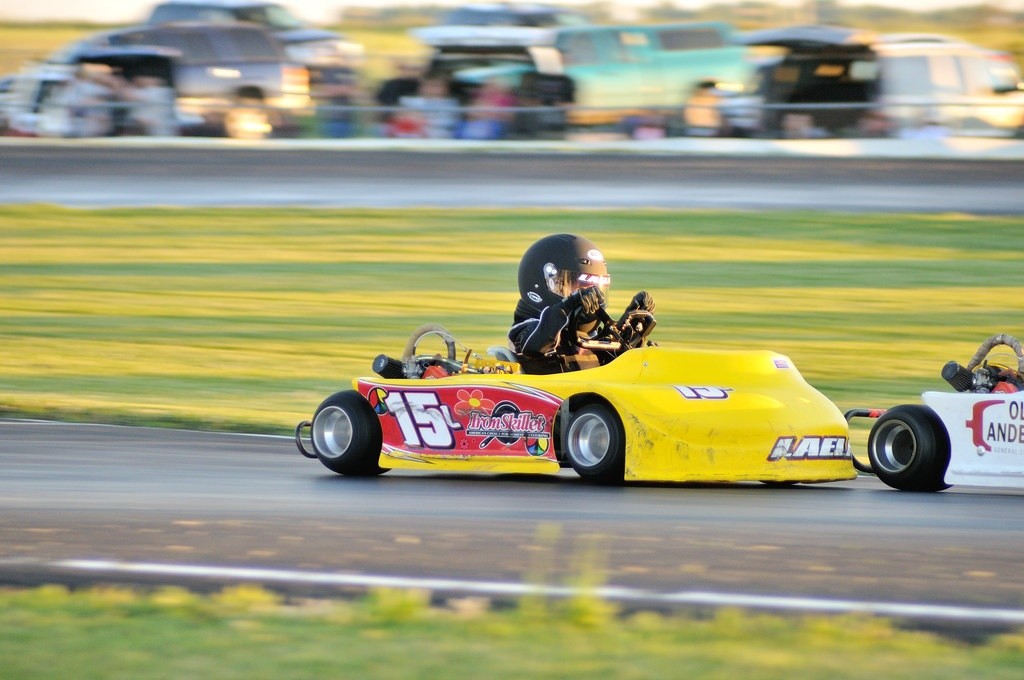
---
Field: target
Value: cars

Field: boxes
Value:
[147,0,367,95]
[51,22,356,140]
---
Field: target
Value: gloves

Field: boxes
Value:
[557,285,605,317]
[616,291,655,331]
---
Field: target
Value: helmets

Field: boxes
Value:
[517,233,610,332]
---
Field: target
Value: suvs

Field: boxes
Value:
[741,25,1024,139]
[449,23,787,129]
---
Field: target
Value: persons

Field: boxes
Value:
[378,68,519,141]
[774,110,956,137]
[38,62,283,137]
[508,234,658,375]
